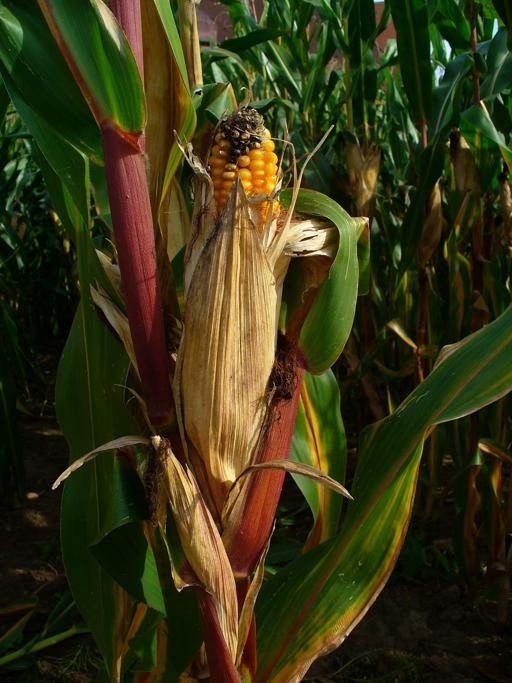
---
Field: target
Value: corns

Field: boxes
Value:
[342,128,512,269]
[174,106,338,526]
